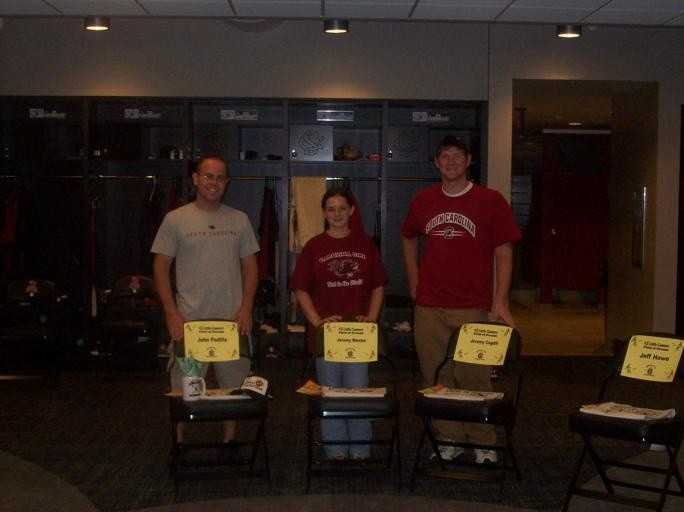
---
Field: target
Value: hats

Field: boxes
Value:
[228,374,275,402]
[436,133,468,150]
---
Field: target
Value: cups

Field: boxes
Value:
[181,376,207,402]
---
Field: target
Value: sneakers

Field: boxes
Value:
[472,446,501,466]
[215,440,247,468]
[428,439,466,463]
[329,450,371,468]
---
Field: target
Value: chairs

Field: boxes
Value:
[0,272,166,380]
[408,318,524,501]
[558,330,684,512]
[169,318,276,492]
[304,318,404,492]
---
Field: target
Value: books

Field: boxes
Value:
[201,377,268,400]
[419,385,504,401]
[579,403,675,422]
[321,386,386,398]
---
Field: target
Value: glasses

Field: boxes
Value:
[198,173,230,182]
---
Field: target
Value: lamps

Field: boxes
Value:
[555,24,583,39]
[324,19,349,34]
[83,15,110,31]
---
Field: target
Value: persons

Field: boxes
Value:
[402,139,519,465]
[151,154,260,470]
[294,188,388,476]
